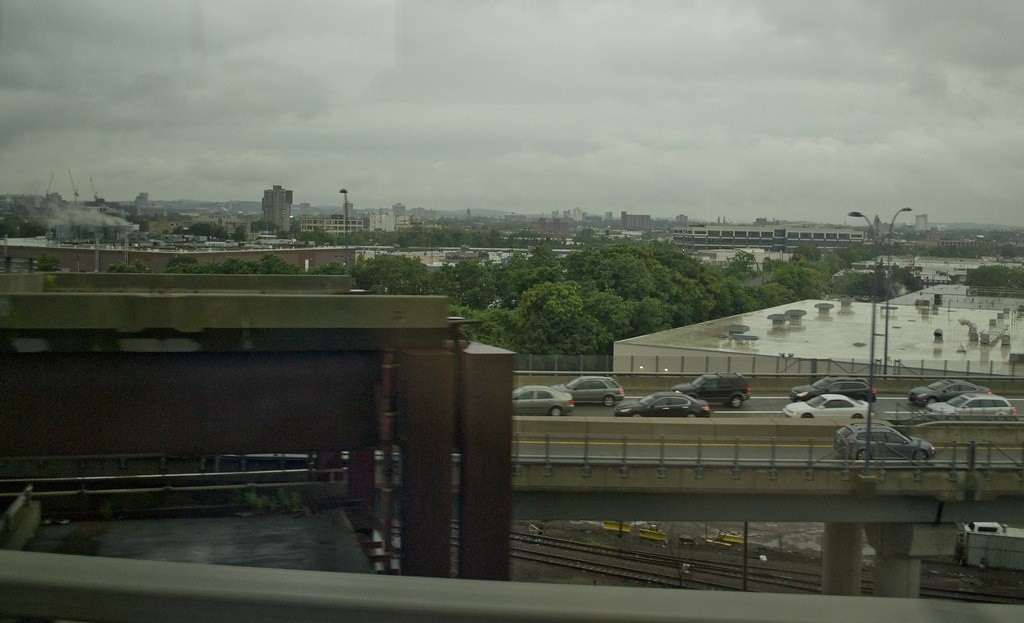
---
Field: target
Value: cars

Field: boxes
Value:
[510,384,575,417]
[907,379,993,406]
[916,392,1017,421]
[614,391,711,419]
[782,394,872,422]
[962,521,1005,538]
[551,375,627,408]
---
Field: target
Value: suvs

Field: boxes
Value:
[789,376,877,405]
[670,370,752,408]
[832,420,937,462]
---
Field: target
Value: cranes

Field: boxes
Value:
[90,176,100,203]
[67,169,80,212]
[44,171,55,199]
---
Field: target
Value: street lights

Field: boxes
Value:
[845,210,879,483]
[340,188,350,267]
[882,204,915,383]
